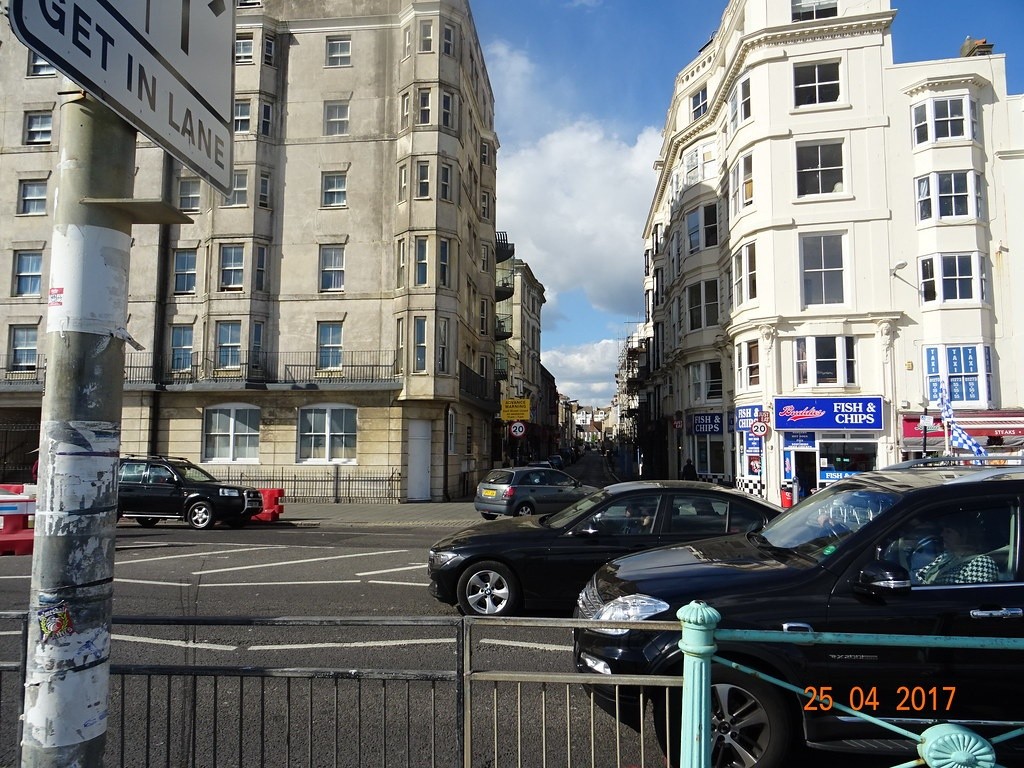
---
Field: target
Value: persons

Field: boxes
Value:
[592,506,651,536]
[597,440,606,456]
[887,514,998,585]
[586,444,594,454]
[682,459,698,482]
[572,446,581,455]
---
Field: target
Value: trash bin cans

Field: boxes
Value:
[781,488,792,508]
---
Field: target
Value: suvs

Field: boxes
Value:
[114,453,265,529]
[568,453,1023,768]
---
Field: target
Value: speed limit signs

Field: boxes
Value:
[511,421,526,438]
[750,421,768,438]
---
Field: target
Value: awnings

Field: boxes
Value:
[952,417,1024,436]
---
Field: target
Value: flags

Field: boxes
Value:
[950,419,988,466]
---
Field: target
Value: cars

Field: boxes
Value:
[526,446,581,472]
[473,466,600,521]
[428,480,848,617]
